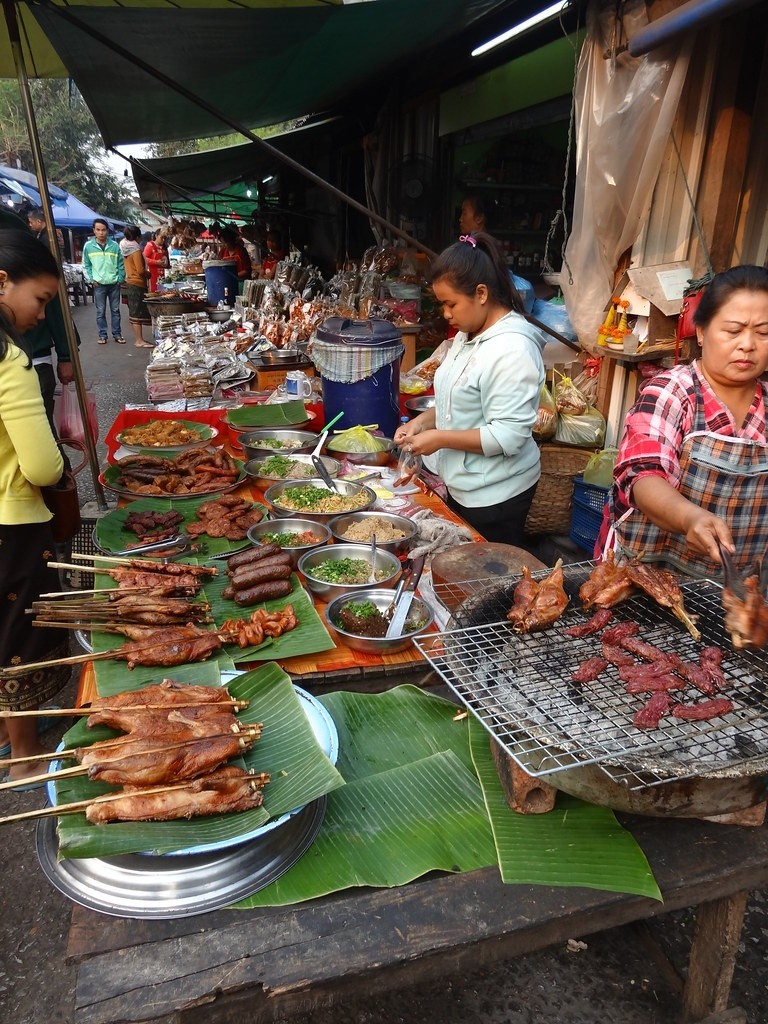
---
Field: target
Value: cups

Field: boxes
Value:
[286,370,312,401]
[623,334,639,354]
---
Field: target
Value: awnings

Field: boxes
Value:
[26,0,562,260]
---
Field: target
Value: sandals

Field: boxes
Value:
[115,336,126,344]
[97,336,106,344]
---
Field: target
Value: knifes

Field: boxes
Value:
[386,556,425,638]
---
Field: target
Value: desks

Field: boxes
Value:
[65,428,768,1024]
[62,263,94,307]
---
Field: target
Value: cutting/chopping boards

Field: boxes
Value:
[430,542,550,613]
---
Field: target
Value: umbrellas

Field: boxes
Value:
[0,163,130,229]
[0,0,345,514]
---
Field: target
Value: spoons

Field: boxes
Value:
[383,568,413,624]
[367,534,378,584]
[301,411,344,448]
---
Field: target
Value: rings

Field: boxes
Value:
[407,446,412,453]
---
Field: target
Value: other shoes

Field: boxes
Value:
[53,552,69,582]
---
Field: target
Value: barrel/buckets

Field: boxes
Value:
[203,259,239,307]
[316,316,408,440]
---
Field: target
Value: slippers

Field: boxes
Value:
[0,707,64,757]
[3,775,47,792]
[135,342,155,348]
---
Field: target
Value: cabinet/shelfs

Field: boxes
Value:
[467,181,558,235]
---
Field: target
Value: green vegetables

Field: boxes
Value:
[263,483,380,630]
[247,438,313,478]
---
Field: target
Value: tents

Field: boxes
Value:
[129,114,367,207]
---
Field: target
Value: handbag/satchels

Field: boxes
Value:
[583,448,621,486]
[52,380,100,451]
[41,438,89,544]
[674,292,704,366]
[529,368,606,447]
[328,423,386,453]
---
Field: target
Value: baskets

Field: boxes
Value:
[567,475,610,555]
[523,447,597,535]
[69,518,108,589]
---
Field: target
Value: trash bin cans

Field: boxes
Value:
[317,316,406,438]
[202,260,238,306]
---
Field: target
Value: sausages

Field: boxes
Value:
[118,447,241,494]
[221,542,292,606]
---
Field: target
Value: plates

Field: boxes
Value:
[46,669,339,858]
[35,792,328,921]
[91,510,275,560]
[98,469,250,502]
[113,445,139,461]
[73,586,315,654]
[113,423,219,453]
[218,410,317,432]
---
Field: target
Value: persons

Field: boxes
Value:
[257,230,286,279]
[121,225,156,347]
[0,201,80,511]
[595,266,768,584]
[80,219,126,344]
[141,228,169,292]
[393,229,547,545]
[447,191,503,339]
[218,225,252,282]
[28,207,81,353]
[0,229,74,792]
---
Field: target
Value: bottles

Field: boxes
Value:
[396,416,409,430]
[488,160,554,283]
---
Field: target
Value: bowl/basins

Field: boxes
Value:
[325,510,418,557]
[604,336,624,350]
[404,395,435,418]
[263,478,377,525]
[236,429,322,460]
[246,518,333,571]
[324,587,435,655]
[174,281,203,293]
[324,434,397,466]
[209,310,233,322]
[297,543,401,603]
[142,298,206,318]
[179,272,205,281]
[260,349,303,366]
[242,454,343,493]
[442,567,768,819]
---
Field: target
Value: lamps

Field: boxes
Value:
[471,0,574,58]
